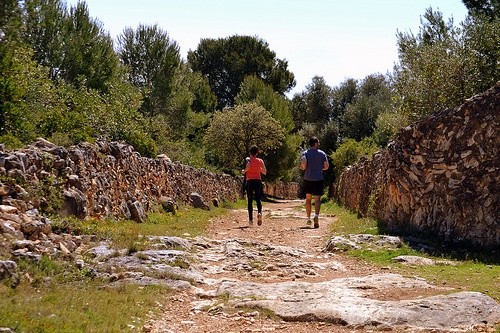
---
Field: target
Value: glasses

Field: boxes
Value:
[318,141,321,143]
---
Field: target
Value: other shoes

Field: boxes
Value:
[314,216,319,228]
[257,213,263,225]
[306,221,312,225]
[249,221,253,225]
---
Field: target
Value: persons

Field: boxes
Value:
[299,137,329,228]
[241,144,267,226]
